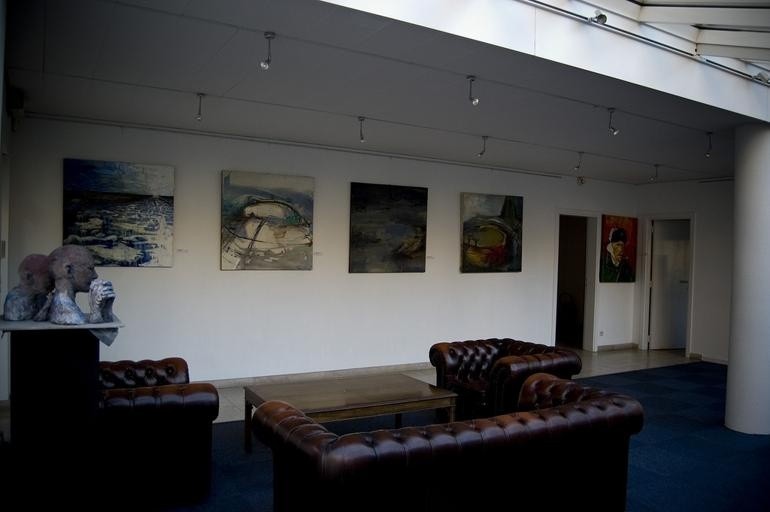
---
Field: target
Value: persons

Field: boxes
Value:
[2,251,55,324]
[600,227,635,282]
[47,244,118,327]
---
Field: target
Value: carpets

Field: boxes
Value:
[211,361,770,512]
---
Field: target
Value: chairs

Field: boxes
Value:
[430,338,582,421]
[10,329,218,511]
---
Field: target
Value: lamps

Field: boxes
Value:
[465,73,482,109]
[568,149,587,187]
[703,131,714,161]
[355,115,370,146]
[255,31,281,74]
[603,105,622,139]
[589,14,608,26]
[476,130,491,159]
[646,164,661,184]
[195,90,212,124]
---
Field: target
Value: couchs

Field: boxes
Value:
[252,372,645,512]
